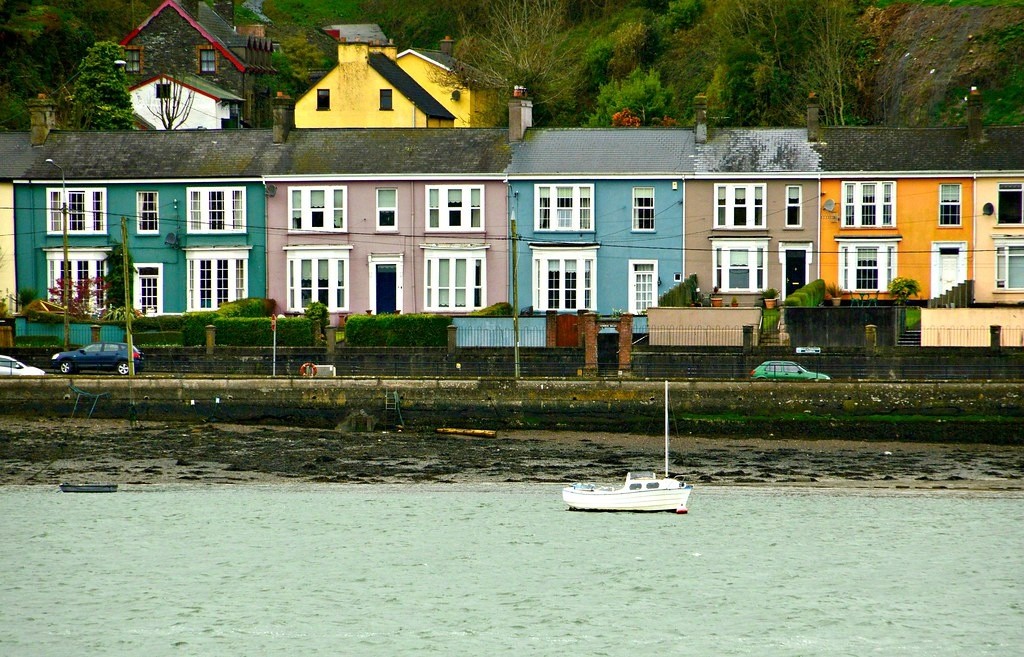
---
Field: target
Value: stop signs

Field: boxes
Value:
[271,314,276,331]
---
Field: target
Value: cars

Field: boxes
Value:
[748,359,830,382]
[0,355,46,375]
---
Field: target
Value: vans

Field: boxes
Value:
[51,341,146,375]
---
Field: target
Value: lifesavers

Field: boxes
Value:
[300,363,317,376]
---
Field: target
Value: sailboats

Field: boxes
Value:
[558,381,698,514]
